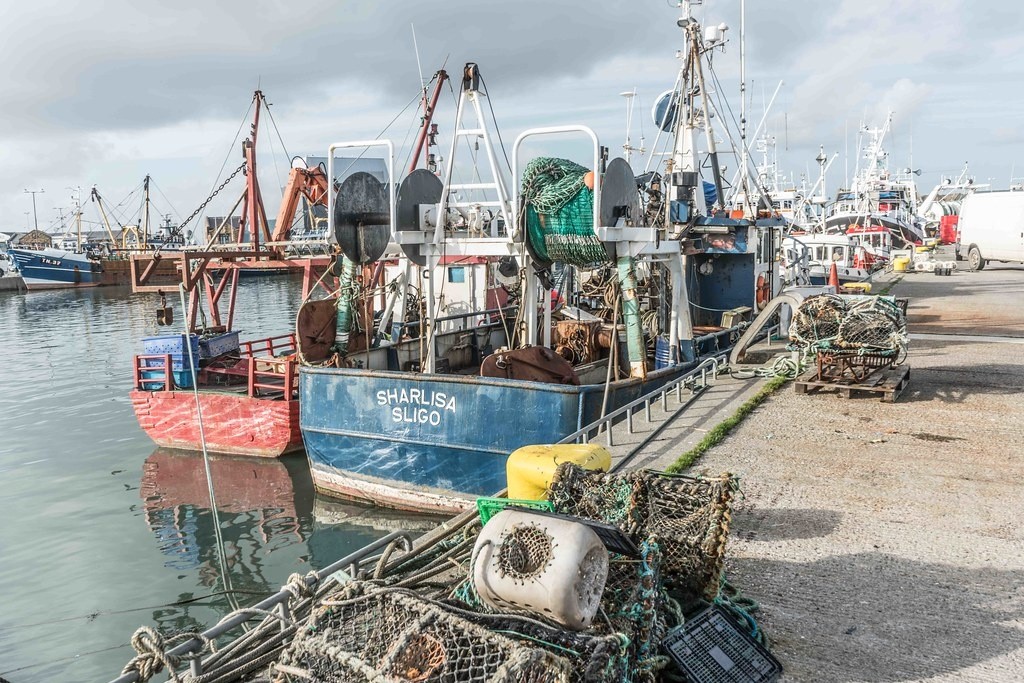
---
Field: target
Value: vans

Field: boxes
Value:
[956,190,1024,271]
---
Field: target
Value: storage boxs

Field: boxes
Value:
[934,268,952,276]
[142,333,201,390]
[198,329,244,358]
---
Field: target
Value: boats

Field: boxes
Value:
[7,186,103,289]
[97,175,185,277]
[130,81,334,463]
[171,75,336,275]
[294,0,782,516]
[731,81,935,286]
